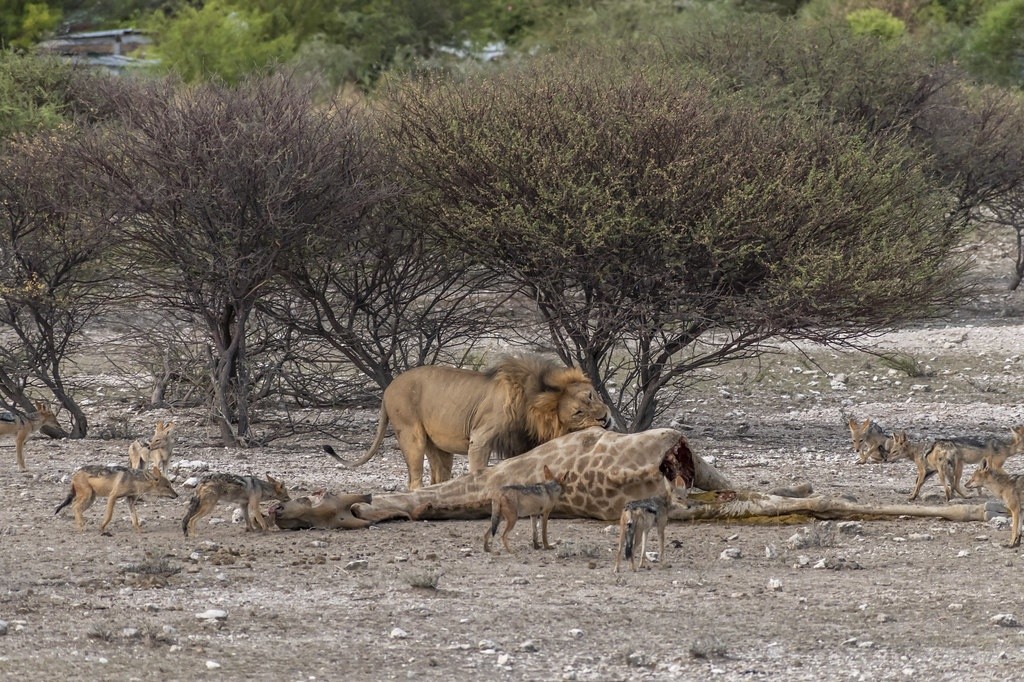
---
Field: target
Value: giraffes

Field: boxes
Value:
[268,426,1010,524]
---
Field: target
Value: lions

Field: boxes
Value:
[319,353,615,490]
